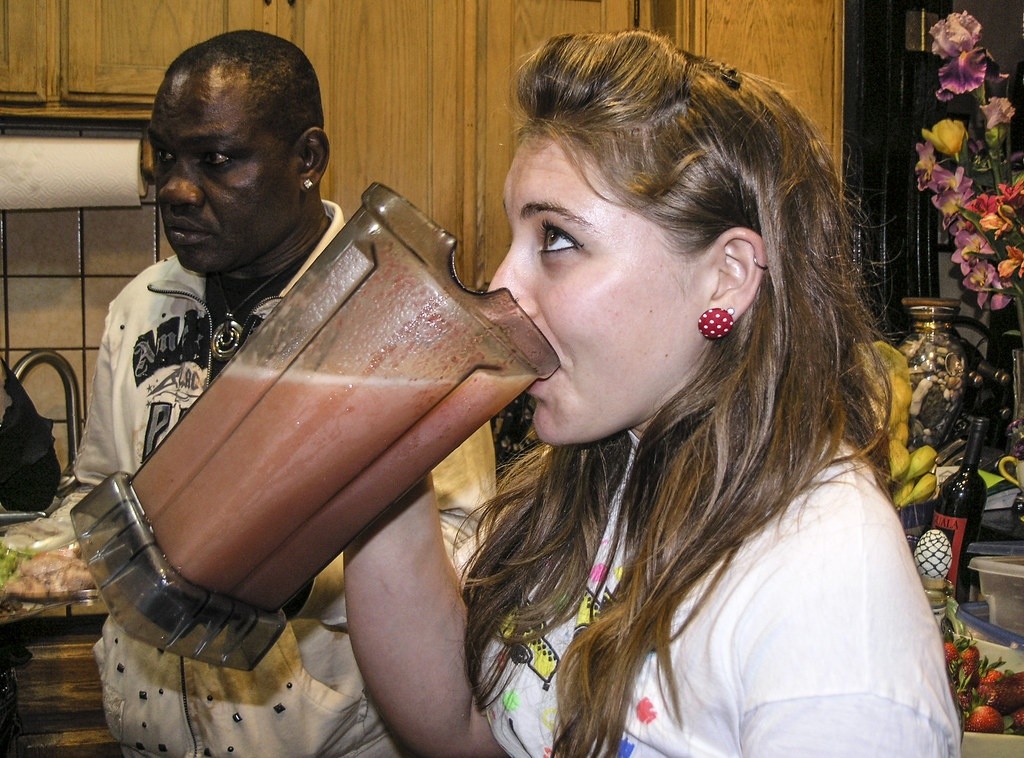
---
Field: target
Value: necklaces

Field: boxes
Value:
[211,265,284,362]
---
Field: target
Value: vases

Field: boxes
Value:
[1012,350,1024,460]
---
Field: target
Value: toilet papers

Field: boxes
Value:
[0,134,149,209]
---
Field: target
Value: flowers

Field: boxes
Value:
[914,10,1024,350]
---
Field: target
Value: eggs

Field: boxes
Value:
[914,529,952,580]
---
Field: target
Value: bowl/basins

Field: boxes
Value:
[952,634,1024,758]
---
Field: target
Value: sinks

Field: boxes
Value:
[0,512,44,539]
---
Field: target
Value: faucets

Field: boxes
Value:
[4,348,82,476]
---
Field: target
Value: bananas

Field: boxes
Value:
[888,440,937,509]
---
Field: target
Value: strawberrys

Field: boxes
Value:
[943,639,1024,736]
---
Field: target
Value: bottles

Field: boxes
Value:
[929,418,987,603]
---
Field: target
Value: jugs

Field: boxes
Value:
[72,183,560,668]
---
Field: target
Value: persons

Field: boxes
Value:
[342,28,965,758]
[72,29,498,758]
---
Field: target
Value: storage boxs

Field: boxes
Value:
[954,540,1024,653]
